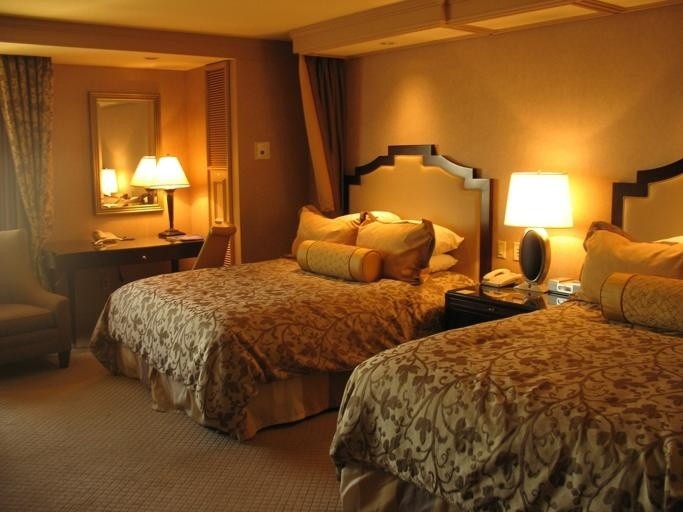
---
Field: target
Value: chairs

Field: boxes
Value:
[177,226,237,272]
[0,229,71,369]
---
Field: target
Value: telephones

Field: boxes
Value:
[92,230,118,243]
[482,268,523,288]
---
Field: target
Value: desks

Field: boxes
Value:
[42,232,205,348]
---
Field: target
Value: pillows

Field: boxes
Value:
[331,211,401,223]
[354,208,434,286]
[282,204,367,260]
[396,219,465,255]
[563,221,683,305]
[600,271,683,334]
[296,239,382,283]
[427,254,458,274]
[652,235,683,244]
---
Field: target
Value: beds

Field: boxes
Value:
[330,158,683,512]
[89,144,493,443]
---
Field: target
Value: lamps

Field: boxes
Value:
[101,167,117,197]
[503,170,573,292]
[149,154,191,237]
[129,155,157,204]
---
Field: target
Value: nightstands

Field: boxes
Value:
[444,283,547,331]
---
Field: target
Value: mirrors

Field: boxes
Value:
[87,91,164,216]
